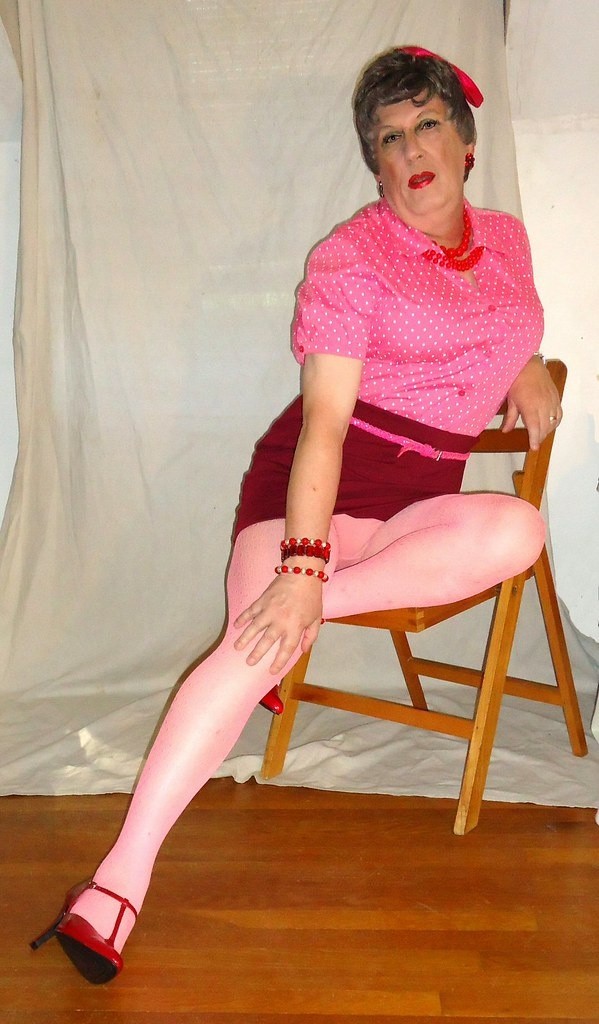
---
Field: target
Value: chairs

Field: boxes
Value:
[261,359,587,834]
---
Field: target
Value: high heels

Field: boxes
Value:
[259,683,283,716]
[29,881,139,985]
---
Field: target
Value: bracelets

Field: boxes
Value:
[280,539,331,564]
[534,352,544,359]
[275,565,330,583]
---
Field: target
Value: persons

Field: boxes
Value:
[29,45,564,984]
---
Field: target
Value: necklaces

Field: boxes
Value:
[422,206,484,275]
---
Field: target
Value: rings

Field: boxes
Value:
[550,416,557,420]
